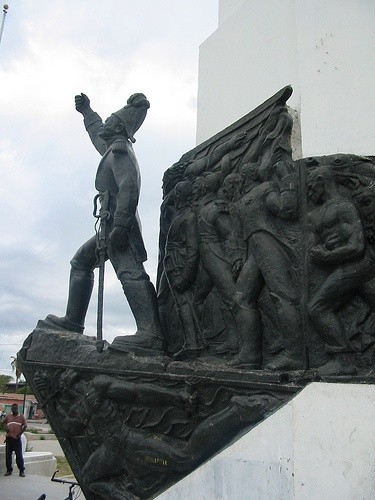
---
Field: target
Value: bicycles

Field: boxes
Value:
[36,470,82,500]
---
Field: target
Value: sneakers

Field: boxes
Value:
[4,471,11,476]
[19,470,25,477]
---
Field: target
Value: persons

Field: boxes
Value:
[26,365,284,500]
[142,106,375,385]
[2,403,29,477]
[44,89,173,359]
